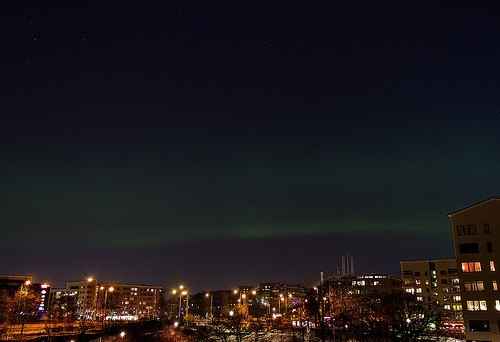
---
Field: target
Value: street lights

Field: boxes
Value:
[172,285,187,318]
[15,280,31,325]
[234,288,242,309]
[279,292,292,313]
[205,292,213,315]
[104,286,114,323]
[87,274,93,324]
